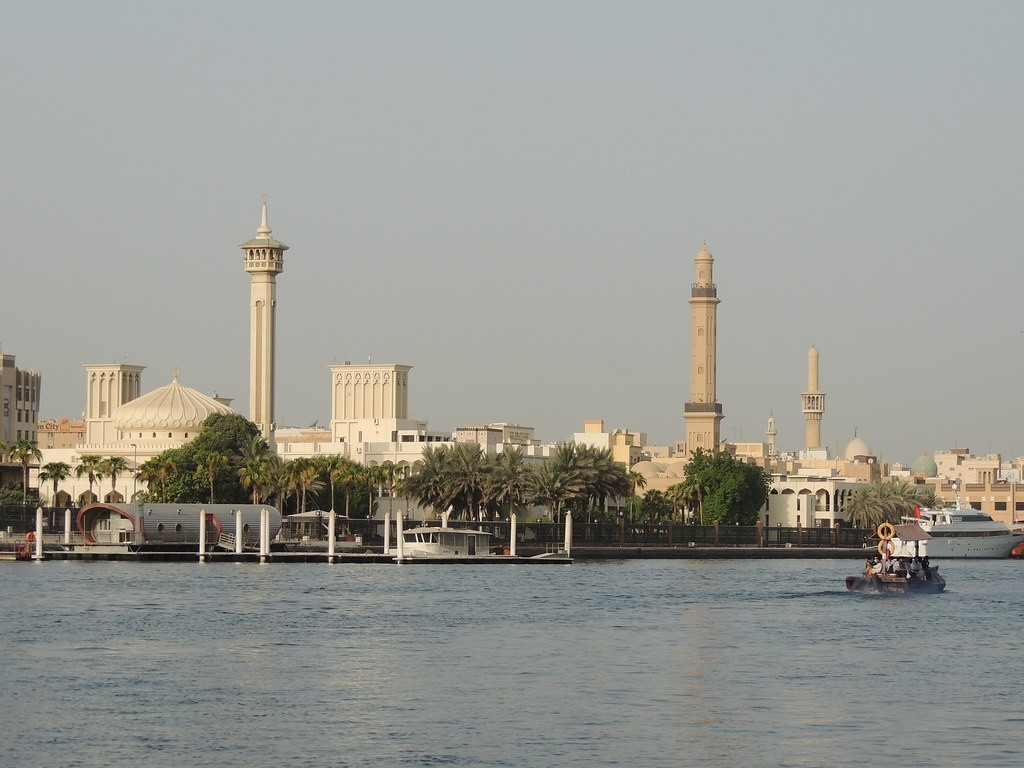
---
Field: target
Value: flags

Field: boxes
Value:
[915,507,920,518]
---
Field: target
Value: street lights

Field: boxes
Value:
[130,443,137,503]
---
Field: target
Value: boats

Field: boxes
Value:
[845,521,946,595]
[880,485,1024,558]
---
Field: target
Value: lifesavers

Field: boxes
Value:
[877,523,897,541]
[877,540,894,555]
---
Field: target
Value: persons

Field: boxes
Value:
[863,536,867,548]
[864,556,933,582]
